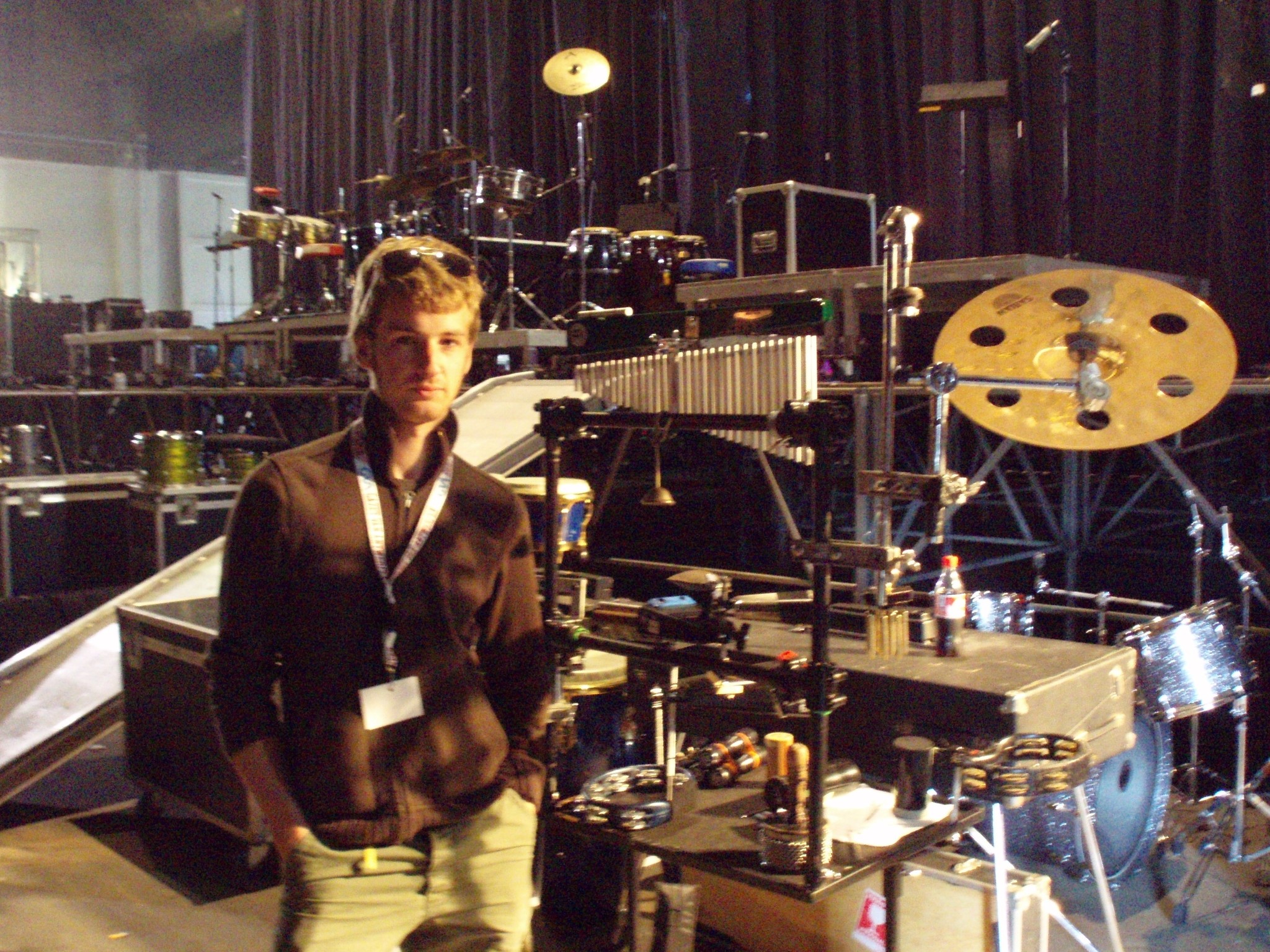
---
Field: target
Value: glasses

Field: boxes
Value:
[382,247,472,277]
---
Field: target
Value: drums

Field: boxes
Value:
[389,208,442,239]
[1107,597,1259,721]
[474,165,541,216]
[285,214,337,254]
[136,431,201,480]
[960,588,1037,640]
[967,706,1177,879]
[341,224,391,277]
[233,211,283,243]
[218,450,270,478]
[564,228,716,301]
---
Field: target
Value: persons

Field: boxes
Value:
[207,238,557,952]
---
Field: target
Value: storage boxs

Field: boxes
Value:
[117,599,270,867]
[736,180,876,278]
[126,484,241,574]
[0,470,150,600]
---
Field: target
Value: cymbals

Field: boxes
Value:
[572,763,697,831]
[932,266,1239,452]
[389,172,452,201]
[353,174,393,185]
[961,731,1094,798]
[411,145,485,165]
[542,48,610,96]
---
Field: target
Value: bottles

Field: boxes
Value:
[932,552,967,655]
